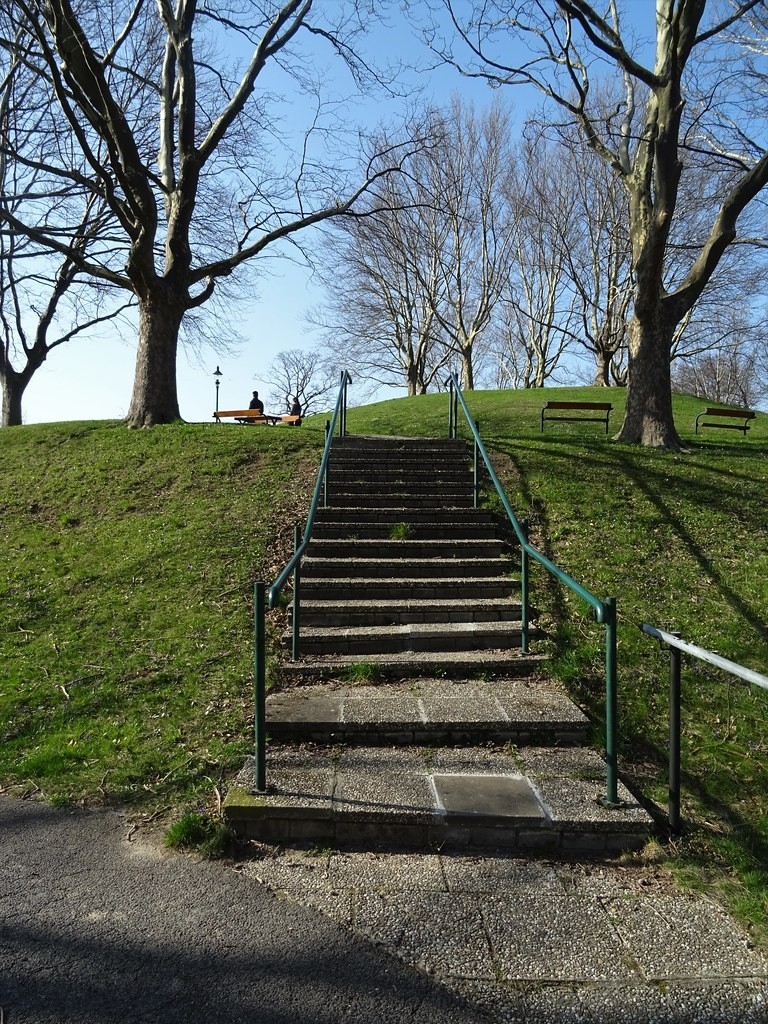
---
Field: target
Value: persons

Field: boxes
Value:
[248,392,263,415]
[290,397,301,427]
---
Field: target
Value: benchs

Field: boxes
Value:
[255,416,299,427]
[691,407,757,435]
[212,409,270,425]
[541,401,614,434]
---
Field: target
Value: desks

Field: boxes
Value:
[234,417,282,425]
